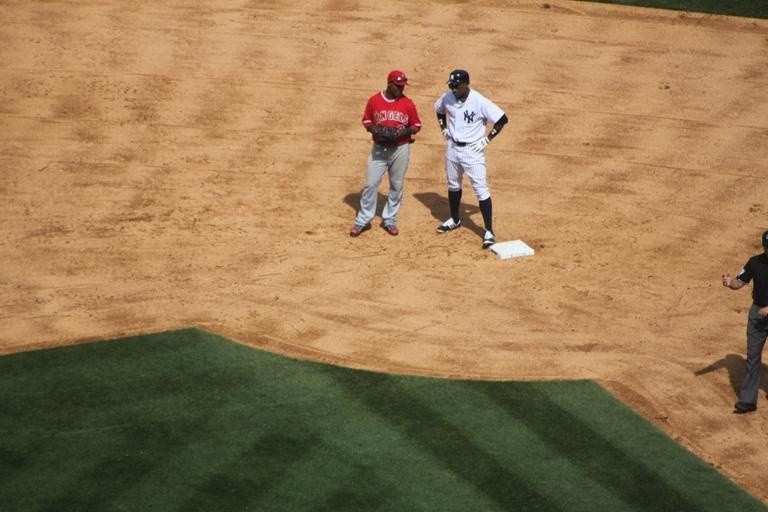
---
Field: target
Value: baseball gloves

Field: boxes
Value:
[373,123,397,143]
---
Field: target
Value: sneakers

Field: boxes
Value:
[385,225,399,236]
[483,228,496,247]
[350,222,371,236]
[436,216,462,234]
[735,402,756,411]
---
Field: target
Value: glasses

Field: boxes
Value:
[392,76,407,82]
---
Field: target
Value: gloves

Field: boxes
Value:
[441,127,452,139]
[469,137,490,153]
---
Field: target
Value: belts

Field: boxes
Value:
[753,300,763,308]
[451,138,467,147]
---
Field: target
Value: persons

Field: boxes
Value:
[722,230,768,412]
[351,70,422,237]
[434,69,509,250]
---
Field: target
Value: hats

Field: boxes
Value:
[762,231,768,246]
[387,70,410,86]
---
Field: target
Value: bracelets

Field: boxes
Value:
[438,120,445,127]
[490,129,499,136]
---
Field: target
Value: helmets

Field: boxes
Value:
[446,69,470,89]
